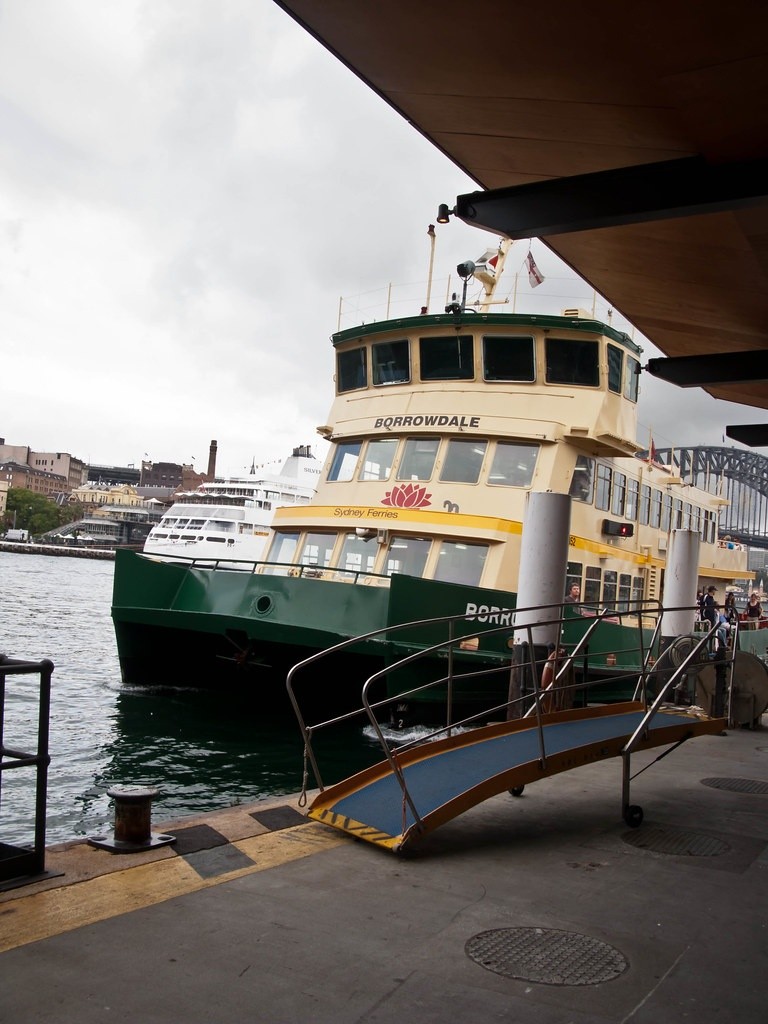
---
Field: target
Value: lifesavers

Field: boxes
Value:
[541,649,576,712]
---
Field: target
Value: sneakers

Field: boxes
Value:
[725,647,730,651]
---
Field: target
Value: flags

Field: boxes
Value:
[192,456,196,460]
[651,438,656,460]
[524,252,545,288]
[145,453,148,457]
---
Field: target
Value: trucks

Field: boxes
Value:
[4,529,29,542]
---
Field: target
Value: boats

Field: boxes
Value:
[108,225,767,717]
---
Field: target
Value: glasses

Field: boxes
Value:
[752,598,756,600]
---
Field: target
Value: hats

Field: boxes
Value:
[728,592,734,596]
[705,586,718,591]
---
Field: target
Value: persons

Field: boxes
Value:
[734,537,741,551]
[695,593,703,622]
[725,592,735,622]
[700,585,718,654]
[741,593,763,630]
[564,582,585,616]
[718,535,734,550]
[712,601,730,651]
